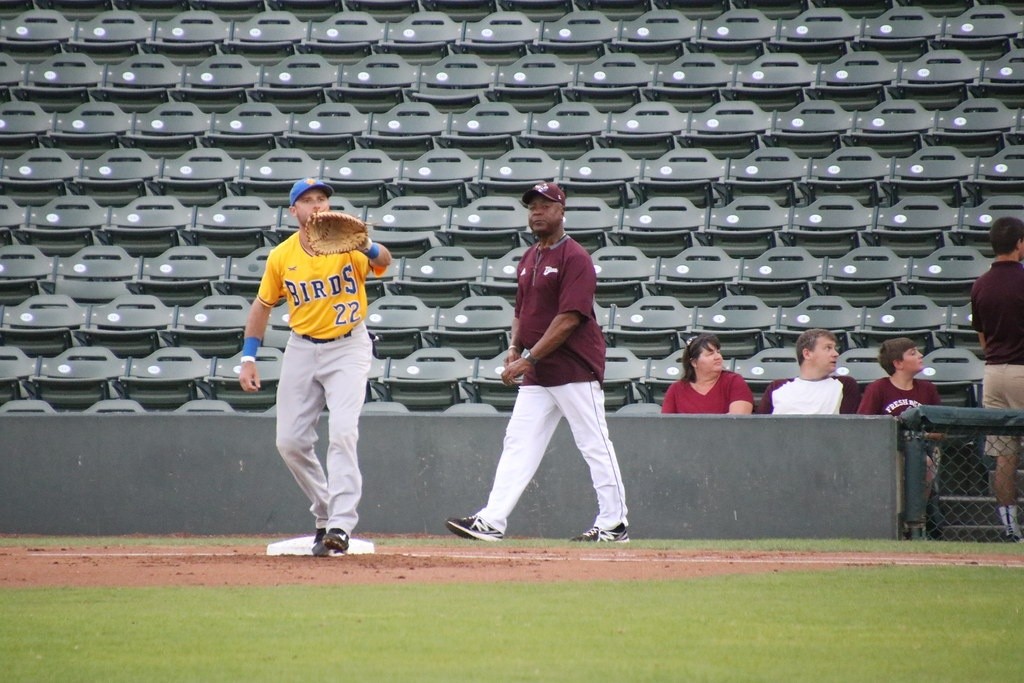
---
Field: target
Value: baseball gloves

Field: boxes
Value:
[305,210,368,254]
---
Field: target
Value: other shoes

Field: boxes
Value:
[1006,534,1024,543]
[902,531,932,541]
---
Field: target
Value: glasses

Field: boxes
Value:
[686,336,699,358]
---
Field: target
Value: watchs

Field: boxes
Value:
[521,348,539,366]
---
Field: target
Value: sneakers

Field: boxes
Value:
[325,528,350,553]
[312,528,330,557]
[445,514,504,542]
[571,523,630,543]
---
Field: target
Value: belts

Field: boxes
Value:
[293,328,353,344]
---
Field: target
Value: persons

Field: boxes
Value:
[857,337,944,541]
[661,332,754,414]
[443,182,630,544]
[756,329,861,414]
[971,217,1024,541]
[239,177,392,555]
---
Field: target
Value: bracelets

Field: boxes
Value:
[509,345,520,351]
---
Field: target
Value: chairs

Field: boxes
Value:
[0,0,1024,489]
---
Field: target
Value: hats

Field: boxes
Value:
[522,182,566,206]
[290,178,333,206]
